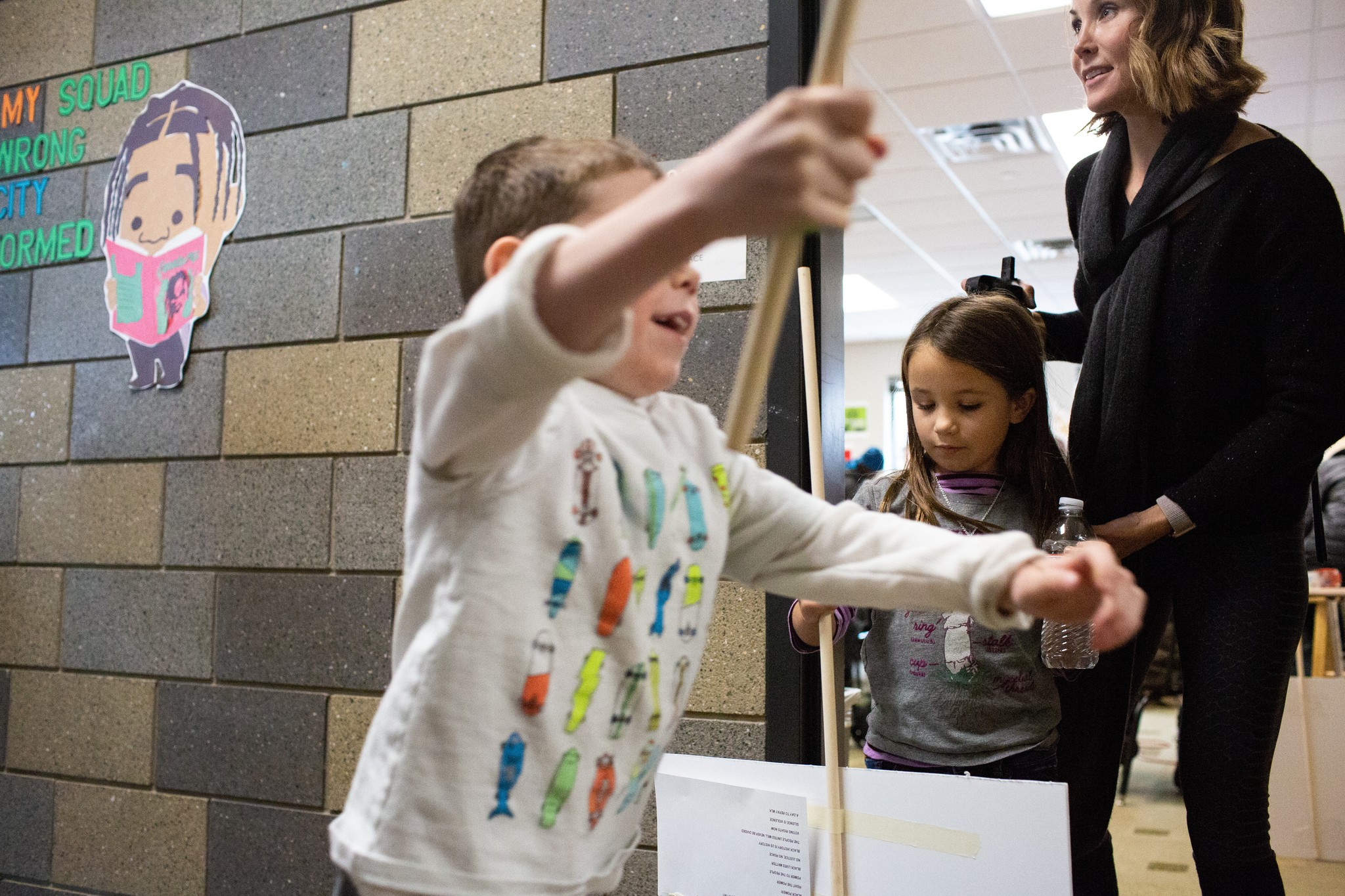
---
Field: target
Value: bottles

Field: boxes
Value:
[1041,497,1099,669]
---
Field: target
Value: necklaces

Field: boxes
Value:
[931,468,1010,533]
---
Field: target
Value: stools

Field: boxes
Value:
[1306,584,1345,678]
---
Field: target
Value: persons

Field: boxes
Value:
[327,86,1147,896]
[788,291,1099,781]
[963,0,1345,896]
